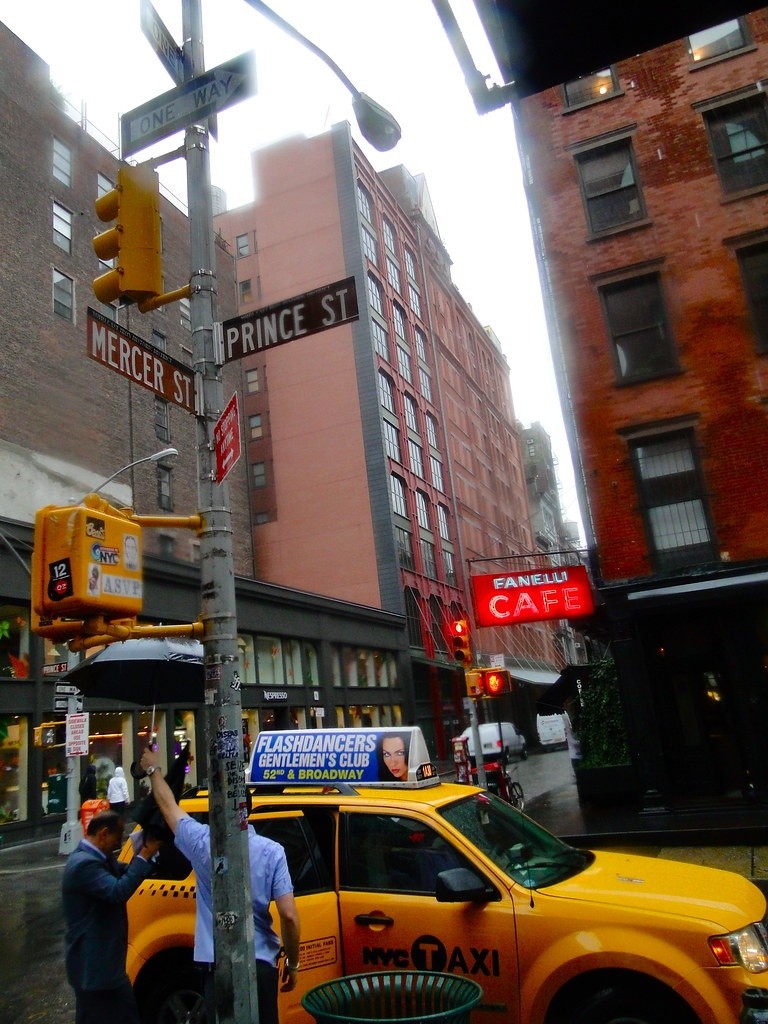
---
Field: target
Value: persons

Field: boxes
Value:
[63,810,161,1024]
[106,767,130,817]
[80,764,97,802]
[377,730,409,783]
[140,747,300,1024]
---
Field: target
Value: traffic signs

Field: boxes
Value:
[141,0,218,139]
[211,389,245,488]
[83,307,197,414]
[39,661,68,675]
[54,681,81,696]
[53,696,83,713]
[115,48,255,162]
[211,275,359,373]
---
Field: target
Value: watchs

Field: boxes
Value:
[146,765,161,776]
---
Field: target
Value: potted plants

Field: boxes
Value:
[563,654,638,806]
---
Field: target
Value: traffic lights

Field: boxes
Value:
[91,176,120,302]
[41,726,55,746]
[450,618,470,664]
[481,670,512,697]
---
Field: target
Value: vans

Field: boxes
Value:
[538,709,572,751]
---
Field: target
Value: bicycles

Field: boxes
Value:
[491,745,525,812]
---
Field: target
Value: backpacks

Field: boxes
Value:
[78,775,92,796]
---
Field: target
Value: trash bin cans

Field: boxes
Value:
[470,762,501,799]
[301,970,486,1024]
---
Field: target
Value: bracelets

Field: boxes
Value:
[285,958,300,970]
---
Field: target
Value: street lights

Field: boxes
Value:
[168,0,405,1020]
[58,444,177,863]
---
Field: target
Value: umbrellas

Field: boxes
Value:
[132,739,189,848]
[58,622,206,779]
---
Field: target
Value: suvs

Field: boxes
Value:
[96,776,767,1024]
[459,722,528,765]
